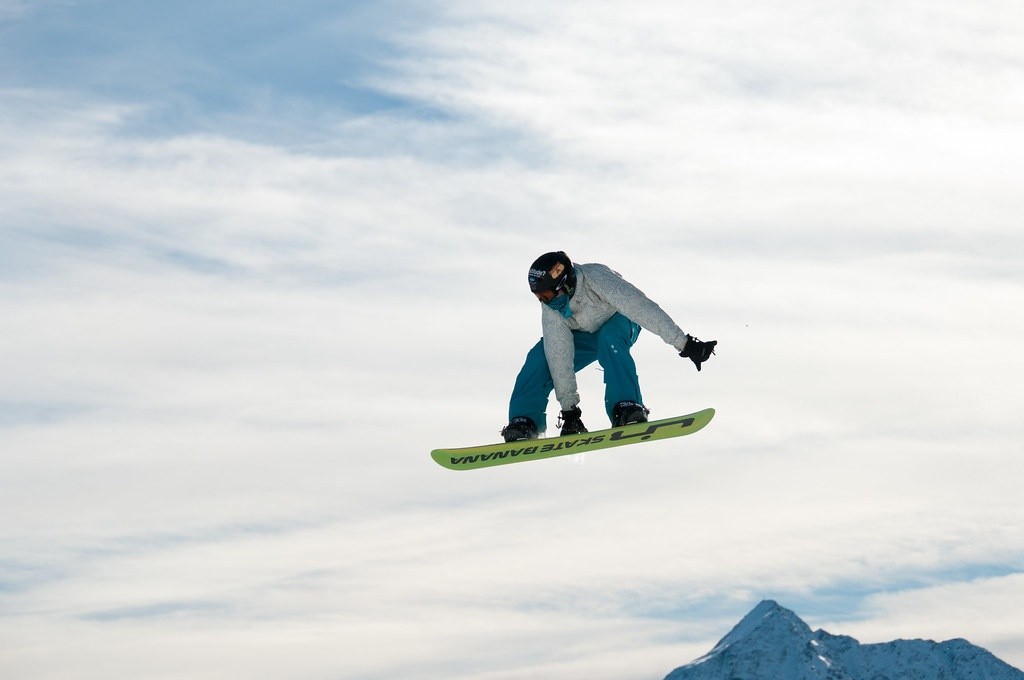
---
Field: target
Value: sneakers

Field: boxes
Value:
[611,401,649,427]
[501,416,539,442]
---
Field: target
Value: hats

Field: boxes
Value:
[529,251,575,293]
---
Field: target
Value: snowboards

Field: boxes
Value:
[430,406,718,469]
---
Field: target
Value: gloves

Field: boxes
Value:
[558,405,588,436]
[679,334,717,372]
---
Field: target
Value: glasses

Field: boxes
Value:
[534,275,568,304]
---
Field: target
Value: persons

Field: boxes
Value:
[502,251,717,443]
